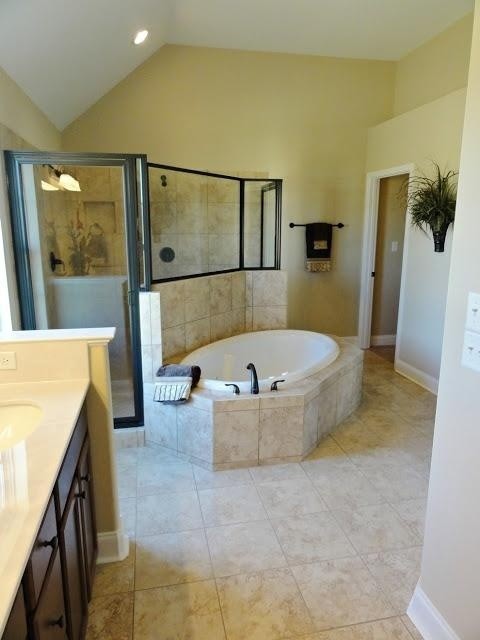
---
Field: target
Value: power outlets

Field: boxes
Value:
[460,291,480,374]
[0,352,15,369]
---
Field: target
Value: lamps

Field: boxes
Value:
[41,163,81,193]
[38,164,64,193]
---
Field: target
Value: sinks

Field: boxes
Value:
[1,398,46,459]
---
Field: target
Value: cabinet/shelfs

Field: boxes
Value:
[2,397,97,639]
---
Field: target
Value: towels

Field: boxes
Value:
[154,363,200,403]
[305,223,333,273]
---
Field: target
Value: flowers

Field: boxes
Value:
[64,210,92,275]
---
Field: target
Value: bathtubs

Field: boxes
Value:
[178,326,340,391]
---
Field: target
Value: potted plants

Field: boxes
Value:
[395,163,456,254]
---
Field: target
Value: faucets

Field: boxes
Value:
[246,362,260,394]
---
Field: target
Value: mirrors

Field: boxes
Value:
[149,163,283,284]
